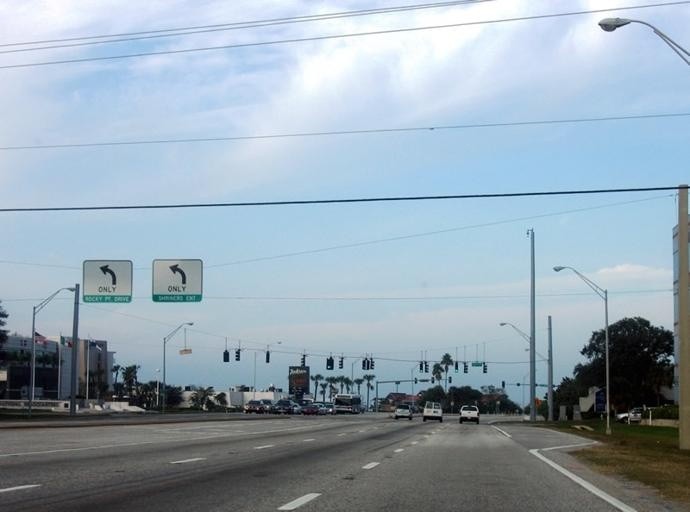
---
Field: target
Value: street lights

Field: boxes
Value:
[523,346,555,422]
[29,287,75,409]
[598,17,690,67]
[499,321,537,420]
[552,262,614,436]
[252,340,283,401]
[163,322,196,411]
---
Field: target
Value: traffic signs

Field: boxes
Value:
[151,258,204,303]
[472,362,482,367]
[82,260,133,304]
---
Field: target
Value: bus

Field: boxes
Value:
[332,393,364,414]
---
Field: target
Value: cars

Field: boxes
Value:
[391,396,483,426]
[244,397,335,416]
[617,407,643,423]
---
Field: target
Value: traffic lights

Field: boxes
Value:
[483,362,488,373]
[235,348,240,361]
[424,361,430,373]
[299,354,377,371]
[464,362,469,373]
[454,362,458,373]
